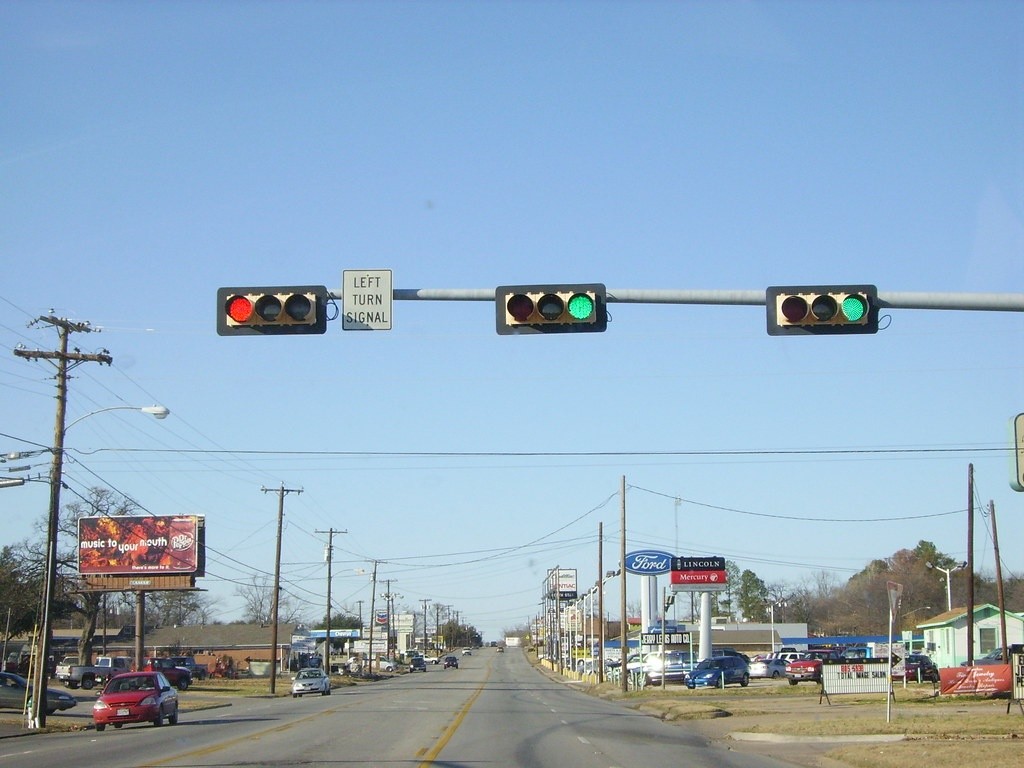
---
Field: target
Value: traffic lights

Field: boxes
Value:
[495,282,607,335]
[767,285,882,335]
[215,285,328,335]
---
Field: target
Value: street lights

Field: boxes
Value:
[899,605,931,634]
[560,569,622,674]
[923,561,967,612]
[29,407,173,730]
[324,569,367,673]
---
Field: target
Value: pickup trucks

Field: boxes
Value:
[54,656,210,692]
[362,655,398,672]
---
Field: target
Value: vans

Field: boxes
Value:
[491,641,496,646]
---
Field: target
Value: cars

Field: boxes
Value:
[93,670,179,729]
[291,667,332,697]
[417,654,440,664]
[748,647,873,685]
[0,672,77,716]
[462,647,471,655]
[960,644,1024,671]
[889,655,940,682]
[682,655,752,688]
[496,646,504,652]
[409,656,427,673]
[472,643,480,649]
[444,657,460,669]
[561,649,749,686]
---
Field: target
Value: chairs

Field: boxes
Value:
[119,683,131,690]
